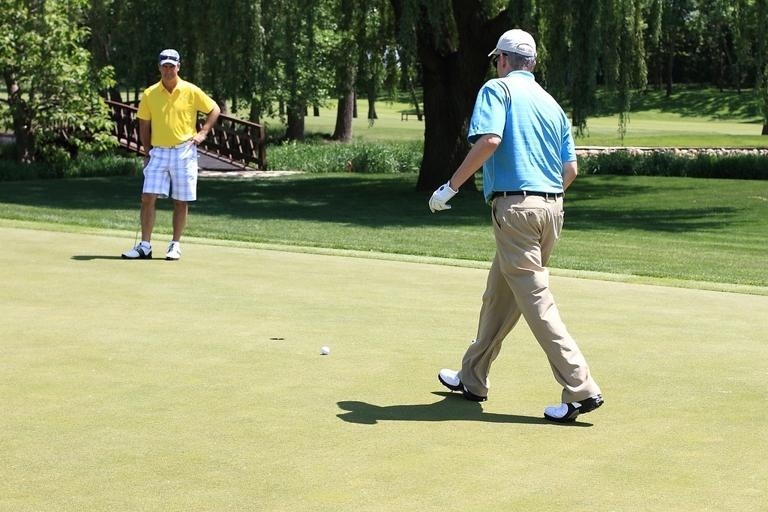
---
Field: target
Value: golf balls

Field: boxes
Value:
[320,344,331,356]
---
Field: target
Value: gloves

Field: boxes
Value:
[428,180,460,214]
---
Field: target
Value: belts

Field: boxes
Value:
[490,190,565,202]
[158,140,193,149]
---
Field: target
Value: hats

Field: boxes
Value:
[159,49,180,67]
[487,28,538,58]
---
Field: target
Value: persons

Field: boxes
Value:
[121,49,221,260]
[428,29,604,422]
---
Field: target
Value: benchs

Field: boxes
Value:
[397,110,424,121]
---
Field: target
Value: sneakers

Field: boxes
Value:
[121,242,153,259]
[438,368,487,402]
[543,392,604,424]
[166,240,181,260]
